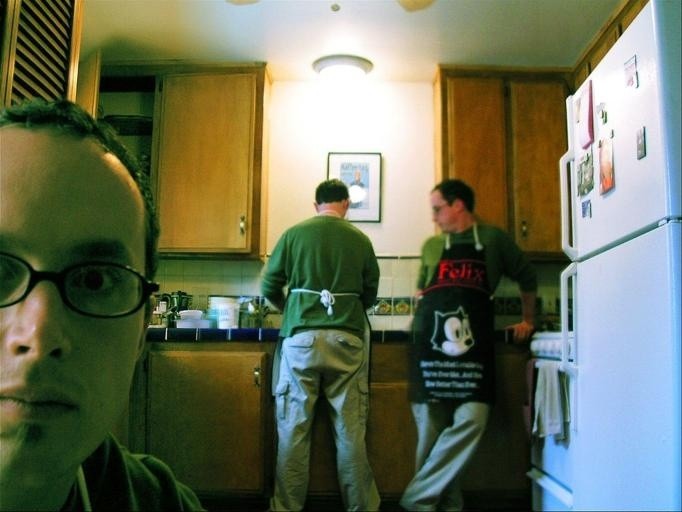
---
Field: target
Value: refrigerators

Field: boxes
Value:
[560,0,680,511]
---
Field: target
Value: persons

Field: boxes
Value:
[0,98,212,512]
[256,178,381,512]
[396,178,537,512]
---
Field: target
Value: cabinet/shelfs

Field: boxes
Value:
[144,349,265,498]
[159,69,256,255]
[441,71,569,257]
[77,50,157,232]
[316,347,418,492]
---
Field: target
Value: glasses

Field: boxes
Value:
[1,249,160,319]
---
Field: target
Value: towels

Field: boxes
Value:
[532,359,570,442]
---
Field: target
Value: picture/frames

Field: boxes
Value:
[326,151,382,222]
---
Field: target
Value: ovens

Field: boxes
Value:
[526,362,572,512]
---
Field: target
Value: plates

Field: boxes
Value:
[208,297,238,322]
